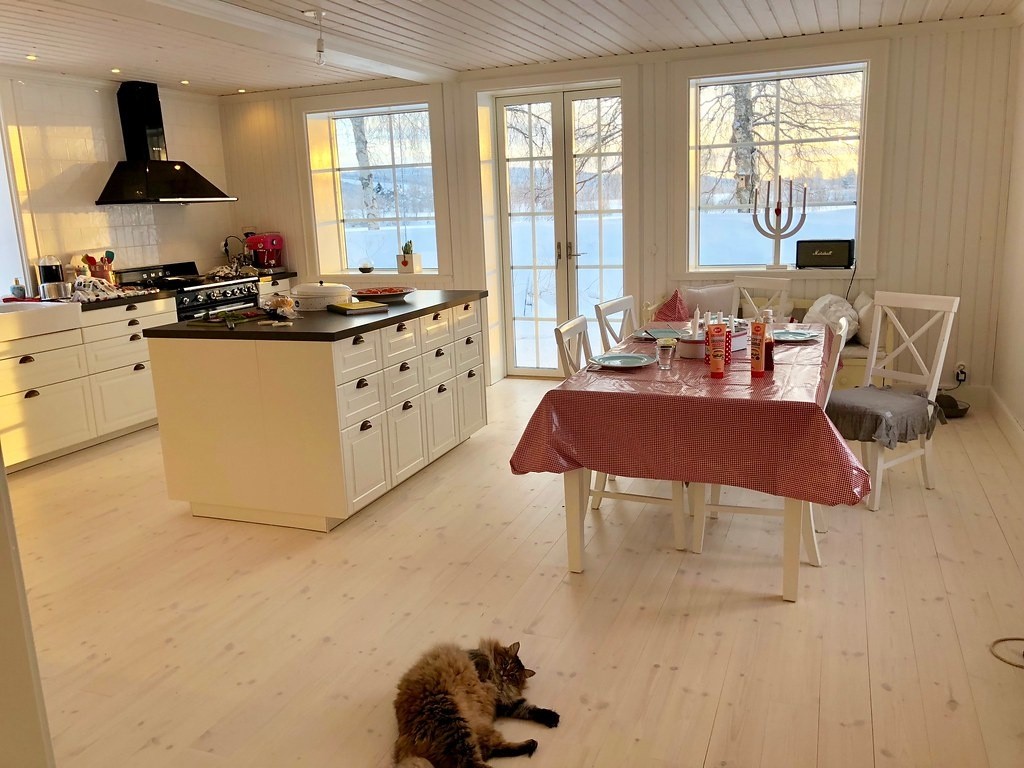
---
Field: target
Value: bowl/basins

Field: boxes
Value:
[359,267,374,273]
[263,308,289,321]
[942,400,970,418]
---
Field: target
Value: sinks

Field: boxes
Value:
[0,301,67,313]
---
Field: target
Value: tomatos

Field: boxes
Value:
[358,287,403,294]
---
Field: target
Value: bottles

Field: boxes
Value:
[10,278,26,299]
[762,309,776,372]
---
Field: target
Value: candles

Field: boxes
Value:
[704,309,711,328]
[693,304,700,330]
[790,180,792,207]
[728,314,735,334]
[717,310,723,324]
[803,188,806,214]
[691,318,698,339]
[766,181,770,207]
[754,189,757,215]
[779,176,781,202]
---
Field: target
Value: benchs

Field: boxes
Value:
[641,297,896,390]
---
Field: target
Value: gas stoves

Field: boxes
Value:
[112,261,260,312]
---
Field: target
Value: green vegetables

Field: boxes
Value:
[216,311,247,320]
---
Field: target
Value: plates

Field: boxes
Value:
[773,329,818,343]
[634,328,690,339]
[352,286,417,303]
[588,353,657,368]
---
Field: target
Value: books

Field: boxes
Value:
[327,301,389,316]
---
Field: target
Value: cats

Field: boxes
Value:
[393,636,561,768]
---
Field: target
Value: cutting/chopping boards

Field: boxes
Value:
[186,310,268,327]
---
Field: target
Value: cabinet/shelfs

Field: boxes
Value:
[0,297,178,474]
[147,279,488,534]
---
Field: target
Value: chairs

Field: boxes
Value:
[731,275,791,323]
[592,295,639,481]
[825,292,960,511]
[554,315,695,551]
[690,316,849,567]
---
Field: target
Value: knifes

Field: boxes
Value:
[226,319,235,330]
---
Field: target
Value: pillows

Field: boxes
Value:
[681,282,734,318]
[802,293,860,342]
[853,290,886,348]
[653,288,689,322]
[741,303,795,321]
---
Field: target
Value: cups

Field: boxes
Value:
[91,271,114,284]
[656,345,675,370]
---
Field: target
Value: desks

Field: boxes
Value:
[509,322,871,602]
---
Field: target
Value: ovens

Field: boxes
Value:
[178,295,258,320]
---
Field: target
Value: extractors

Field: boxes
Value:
[95,80,239,205]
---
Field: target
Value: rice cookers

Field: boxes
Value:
[245,231,284,269]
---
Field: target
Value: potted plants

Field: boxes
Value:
[396,240,421,273]
